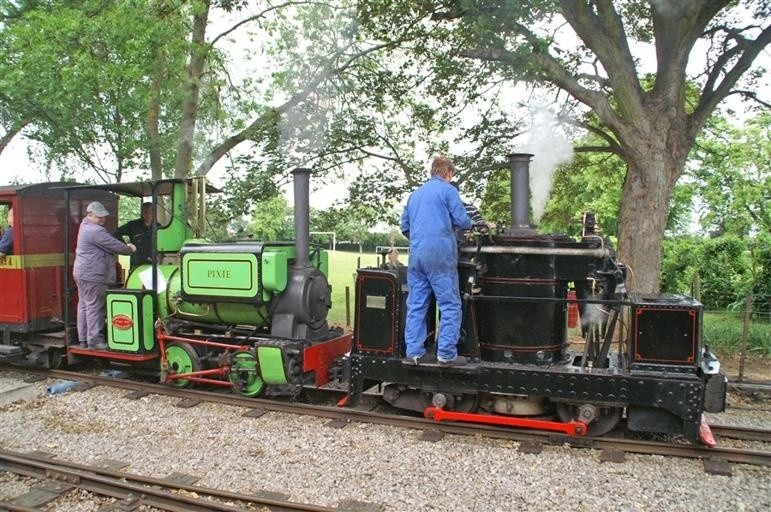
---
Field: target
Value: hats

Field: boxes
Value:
[87,201,110,217]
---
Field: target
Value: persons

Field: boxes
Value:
[0,207,14,256]
[402,157,491,367]
[71,202,154,348]
[387,248,403,265]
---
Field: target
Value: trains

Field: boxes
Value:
[0,151,731,455]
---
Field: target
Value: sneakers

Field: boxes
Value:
[402,354,467,367]
[80,342,108,349]
